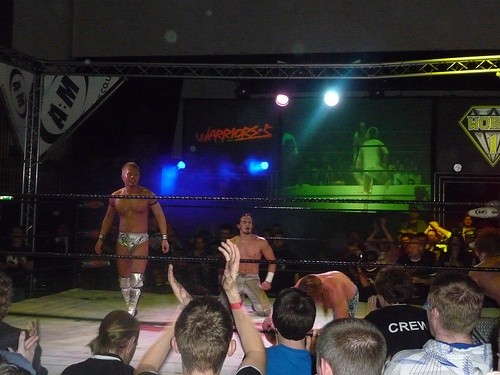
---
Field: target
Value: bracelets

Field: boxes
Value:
[162,234,168,239]
[99,233,105,240]
[230,299,244,310]
[265,271,274,283]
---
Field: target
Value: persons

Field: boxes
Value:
[294,271,359,321]
[151,224,300,297]
[351,121,392,185]
[95,162,169,316]
[387,155,430,185]
[62,239,316,375]
[0,270,48,375]
[282,134,349,187]
[469,226,500,305]
[346,208,480,305]
[315,273,492,375]
[0,225,37,303]
[218,213,277,317]
